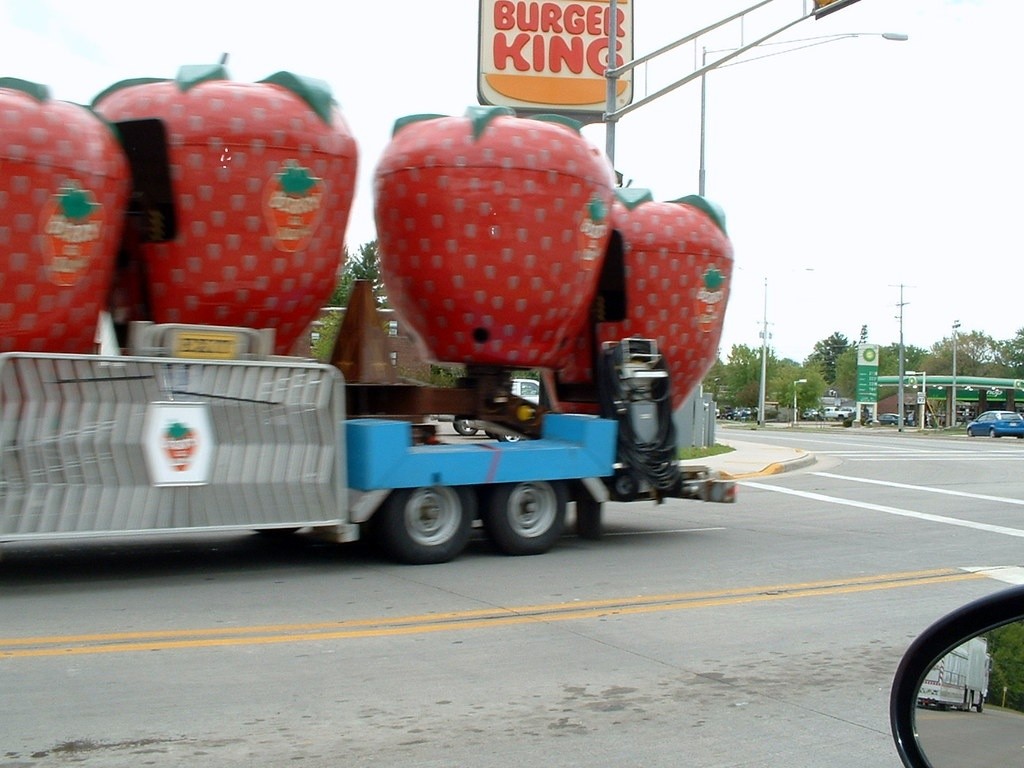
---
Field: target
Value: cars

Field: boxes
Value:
[715,404,759,422]
[865,411,907,426]
[801,405,857,422]
[965,409,1024,439]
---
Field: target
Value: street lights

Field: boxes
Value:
[792,378,808,426]
[694,29,908,196]
[951,319,961,427]
[734,265,815,427]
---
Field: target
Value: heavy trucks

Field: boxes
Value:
[0,328,744,566]
[915,634,995,714]
